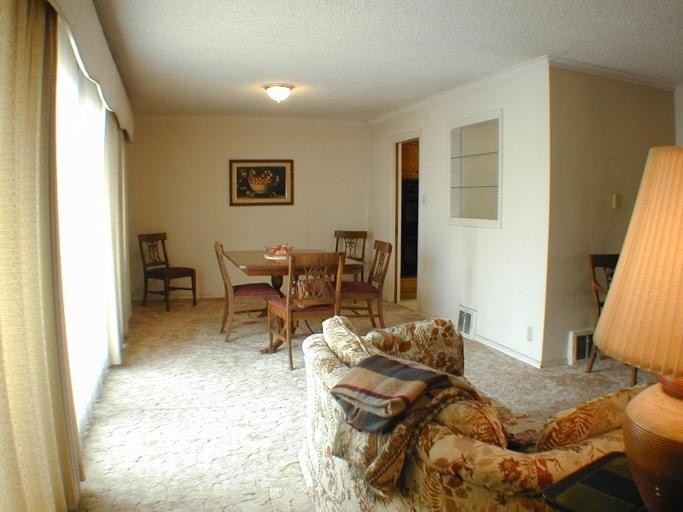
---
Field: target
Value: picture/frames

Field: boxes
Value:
[229,159,294,205]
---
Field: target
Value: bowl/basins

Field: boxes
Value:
[263,253,294,259]
[250,184,272,193]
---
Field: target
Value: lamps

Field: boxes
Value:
[263,82,294,105]
[593,147,683,512]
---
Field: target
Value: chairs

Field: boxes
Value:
[334,230,368,283]
[585,253,638,388]
[137,233,196,311]
[267,252,346,369]
[214,241,281,342]
[297,316,657,511]
[331,239,392,328]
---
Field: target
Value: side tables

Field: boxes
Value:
[542,452,649,512]
